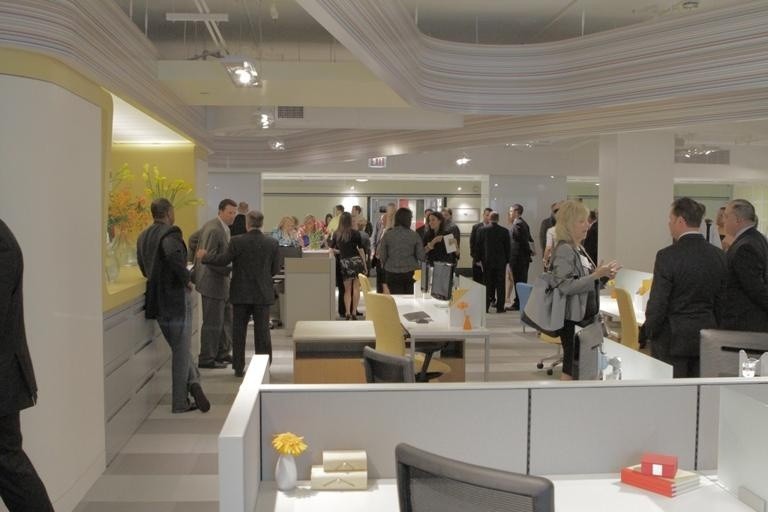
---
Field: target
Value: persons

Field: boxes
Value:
[469,200,624,379]
[137,198,210,413]
[187,199,235,368]
[723,200,768,333]
[716,207,726,241]
[640,197,730,379]
[230,201,249,237]
[196,210,281,376]
[273,205,460,320]
[0,217,54,512]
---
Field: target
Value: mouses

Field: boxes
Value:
[417,319,428,324]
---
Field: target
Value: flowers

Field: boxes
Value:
[106,161,208,243]
[269,428,311,459]
[301,213,328,250]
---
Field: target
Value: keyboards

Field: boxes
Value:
[403,311,432,322]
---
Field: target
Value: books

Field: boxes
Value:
[621,464,699,499]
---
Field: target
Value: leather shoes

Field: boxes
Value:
[199,355,243,377]
[186,383,209,411]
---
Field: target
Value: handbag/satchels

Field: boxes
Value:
[521,272,566,337]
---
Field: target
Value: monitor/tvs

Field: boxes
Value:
[420,261,429,294]
[571,321,616,381]
[279,245,302,270]
[430,261,454,301]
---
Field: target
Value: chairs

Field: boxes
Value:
[614,287,640,350]
[362,344,416,384]
[514,280,563,376]
[356,269,372,296]
[394,441,557,511]
[362,290,453,382]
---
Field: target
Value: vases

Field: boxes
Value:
[309,236,320,250]
[105,229,138,283]
[273,453,299,493]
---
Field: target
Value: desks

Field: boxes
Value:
[257,464,759,511]
[275,248,340,339]
[595,291,649,345]
[289,292,491,384]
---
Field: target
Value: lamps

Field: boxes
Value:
[221,1,264,90]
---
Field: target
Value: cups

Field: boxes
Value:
[741,357,760,378]
[423,287,432,298]
